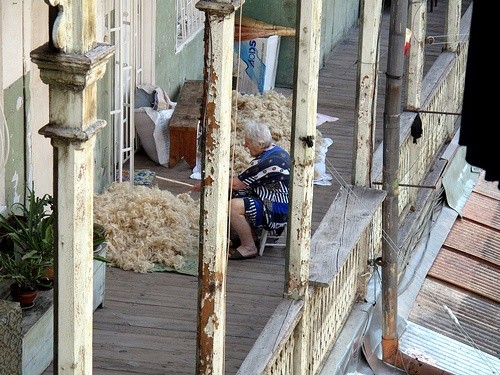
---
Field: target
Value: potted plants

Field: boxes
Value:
[0,180,67,310]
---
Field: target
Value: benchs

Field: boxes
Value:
[169,79,205,169]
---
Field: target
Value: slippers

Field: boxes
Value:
[229,249,258,260]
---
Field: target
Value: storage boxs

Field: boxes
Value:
[232,33,283,96]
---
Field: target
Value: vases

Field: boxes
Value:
[93,224,105,251]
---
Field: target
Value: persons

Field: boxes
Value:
[193,122,292,260]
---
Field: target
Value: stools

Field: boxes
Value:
[261,223,288,256]
